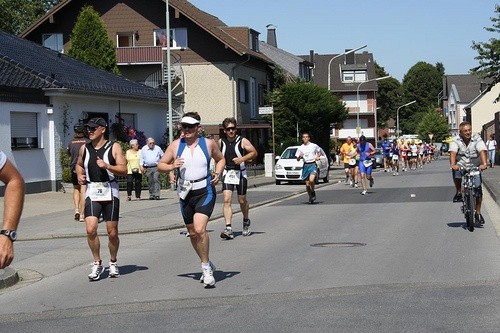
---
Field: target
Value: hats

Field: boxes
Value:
[84,118,107,128]
[180,117,200,126]
[74,123,84,133]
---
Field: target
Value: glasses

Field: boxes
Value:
[226,127,236,131]
[150,143,155,145]
[182,124,195,129]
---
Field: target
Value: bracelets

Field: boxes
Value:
[216,173,220,177]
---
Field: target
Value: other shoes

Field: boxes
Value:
[126,197,131,201]
[345,176,349,182]
[350,178,358,188]
[370,178,374,187]
[384,168,408,176]
[136,198,140,201]
[149,196,160,200]
[362,189,367,195]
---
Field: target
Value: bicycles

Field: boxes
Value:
[452,166,487,232]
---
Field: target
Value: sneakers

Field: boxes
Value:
[307,191,316,204]
[88,260,105,280]
[242,219,251,236]
[220,228,234,239]
[201,262,217,288]
[74,211,80,221]
[109,260,121,278]
[80,216,85,222]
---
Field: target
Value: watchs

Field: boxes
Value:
[0,229,17,242]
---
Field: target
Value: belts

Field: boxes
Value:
[143,164,157,168]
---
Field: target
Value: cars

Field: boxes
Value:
[274,145,330,185]
[370,148,384,168]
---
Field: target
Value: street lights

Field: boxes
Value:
[357,75,393,142]
[328,44,368,93]
[397,99,417,139]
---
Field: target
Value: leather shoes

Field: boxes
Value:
[475,212,485,224]
[453,193,462,202]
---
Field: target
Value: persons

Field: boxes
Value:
[486,135,497,168]
[140,137,164,200]
[75,117,128,281]
[218,117,258,240]
[346,135,377,195]
[66,122,92,222]
[169,131,216,190]
[157,112,226,288]
[331,145,341,166]
[448,122,489,224]
[125,139,142,201]
[295,132,322,204]
[381,138,446,176]
[0,151,25,269]
[340,136,360,188]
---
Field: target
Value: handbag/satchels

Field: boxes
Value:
[132,168,139,175]
